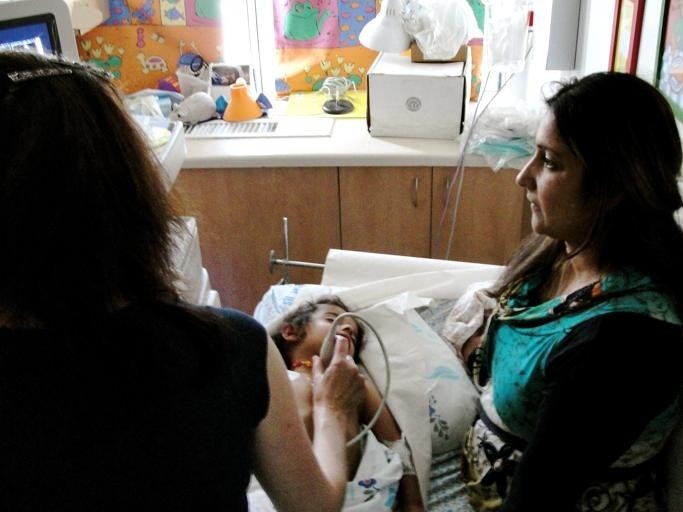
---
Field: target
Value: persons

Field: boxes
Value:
[274,296,423,512]
[462,72,683,512]
[1,53,363,512]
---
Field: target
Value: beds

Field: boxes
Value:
[243,283,474,512]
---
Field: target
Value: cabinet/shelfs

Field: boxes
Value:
[165,166,339,319]
[339,166,526,267]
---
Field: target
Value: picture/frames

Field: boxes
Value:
[652,0,683,125]
[608,0,644,75]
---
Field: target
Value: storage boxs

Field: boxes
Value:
[365,44,472,141]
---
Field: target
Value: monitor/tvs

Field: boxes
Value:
[0,0,81,66]
